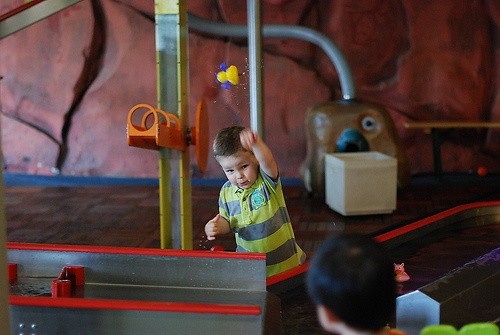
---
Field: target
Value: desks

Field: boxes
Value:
[402,120,500,179]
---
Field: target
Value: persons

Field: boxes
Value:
[308,230,407,335]
[204,126,307,278]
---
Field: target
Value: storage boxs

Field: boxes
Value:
[325,152,397,216]
